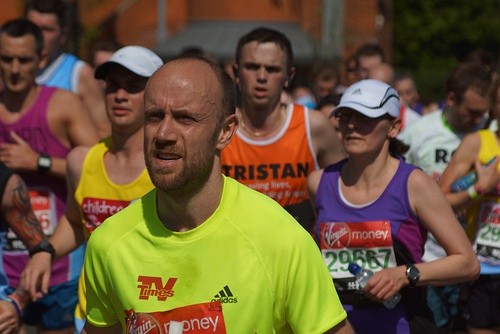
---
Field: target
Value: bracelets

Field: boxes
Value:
[467,184,485,202]
[4,295,24,323]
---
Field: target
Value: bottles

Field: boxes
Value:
[348,263,401,310]
[451,159,493,193]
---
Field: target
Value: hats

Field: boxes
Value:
[94,45,164,80]
[329,79,401,118]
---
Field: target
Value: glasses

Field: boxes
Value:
[335,110,373,123]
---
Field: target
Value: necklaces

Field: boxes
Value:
[231,103,285,138]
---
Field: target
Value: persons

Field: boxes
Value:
[389,63,493,333]
[0,159,51,334]
[179,44,439,142]
[1,16,102,334]
[23,0,113,142]
[436,65,499,334]
[18,46,165,302]
[306,79,482,334]
[74,54,356,334]
[87,39,122,93]
[215,27,351,238]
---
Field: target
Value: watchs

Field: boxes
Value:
[29,239,55,260]
[37,154,51,173]
